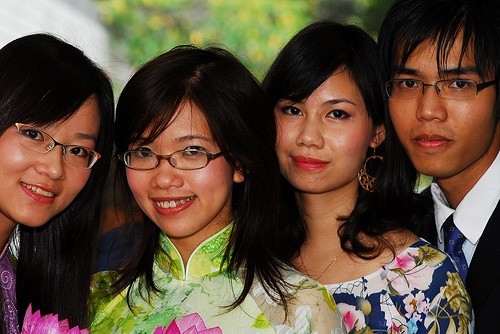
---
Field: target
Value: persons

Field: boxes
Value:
[94,173,153,279]
[0,33,113,334]
[258,19,475,334]
[375,0,500,334]
[85,44,347,334]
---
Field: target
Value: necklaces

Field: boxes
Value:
[299,236,356,286]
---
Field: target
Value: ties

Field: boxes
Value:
[443,215,469,285]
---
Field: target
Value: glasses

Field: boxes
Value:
[13,122,104,170]
[385,79,497,100]
[124,147,223,170]
